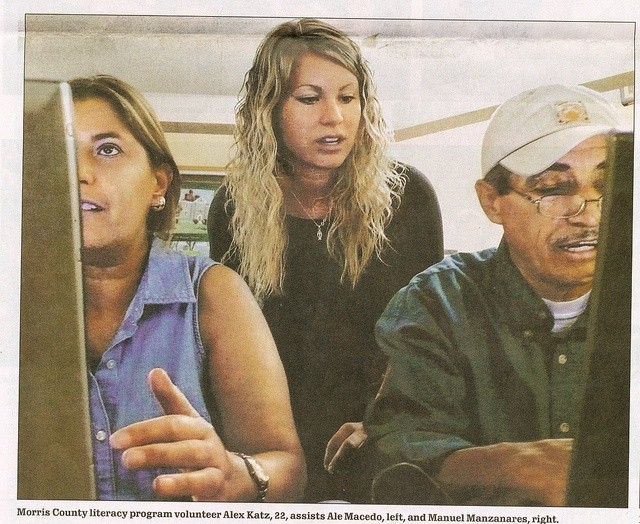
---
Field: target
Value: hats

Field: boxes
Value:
[481,84,633,180]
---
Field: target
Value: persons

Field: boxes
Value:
[208,18,441,503]
[359,82,634,507]
[66,75,308,503]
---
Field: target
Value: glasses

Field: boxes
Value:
[493,182,607,219]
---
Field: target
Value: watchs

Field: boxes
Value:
[231,452,270,503]
